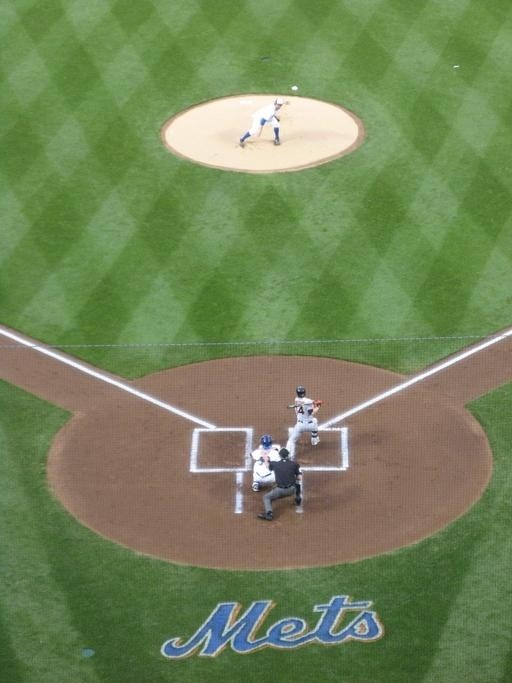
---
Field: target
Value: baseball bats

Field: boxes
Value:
[287,403,312,408]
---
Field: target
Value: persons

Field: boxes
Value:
[238,97,291,146]
[257,449,307,520]
[285,386,322,457]
[251,432,286,486]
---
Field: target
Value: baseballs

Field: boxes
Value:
[292,86,298,91]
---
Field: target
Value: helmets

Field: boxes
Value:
[296,386,308,398]
[261,434,273,449]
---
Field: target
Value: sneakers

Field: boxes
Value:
[293,503,304,514]
[251,481,261,493]
[273,138,281,146]
[311,436,322,446]
[239,138,245,150]
[257,511,273,521]
